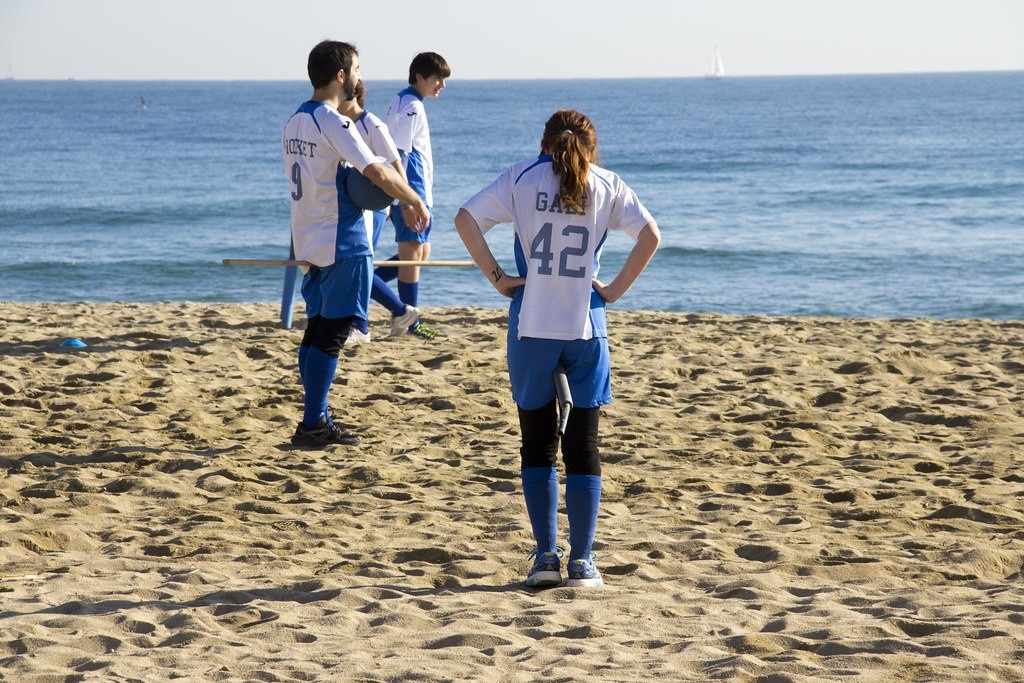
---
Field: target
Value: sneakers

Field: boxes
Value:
[525,546,564,586]
[566,553,604,587]
[344,327,370,346]
[390,304,420,335]
[291,418,360,447]
[409,324,449,343]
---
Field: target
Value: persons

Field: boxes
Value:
[337,79,422,343]
[373,52,451,341]
[453,108,662,586]
[281,38,432,449]
